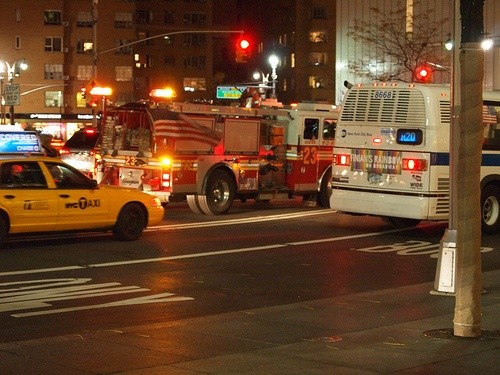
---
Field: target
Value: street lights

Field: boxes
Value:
[0,57,27,125]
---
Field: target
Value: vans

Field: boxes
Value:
[330,80,500,233]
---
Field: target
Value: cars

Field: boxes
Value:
[1,131,165,243]
[60,126,102,172]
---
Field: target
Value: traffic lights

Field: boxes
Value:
[81,88,86,99]
[239,39,250,49]
[419,68,428,77]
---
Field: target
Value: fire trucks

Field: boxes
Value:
[100,99,340,216]
